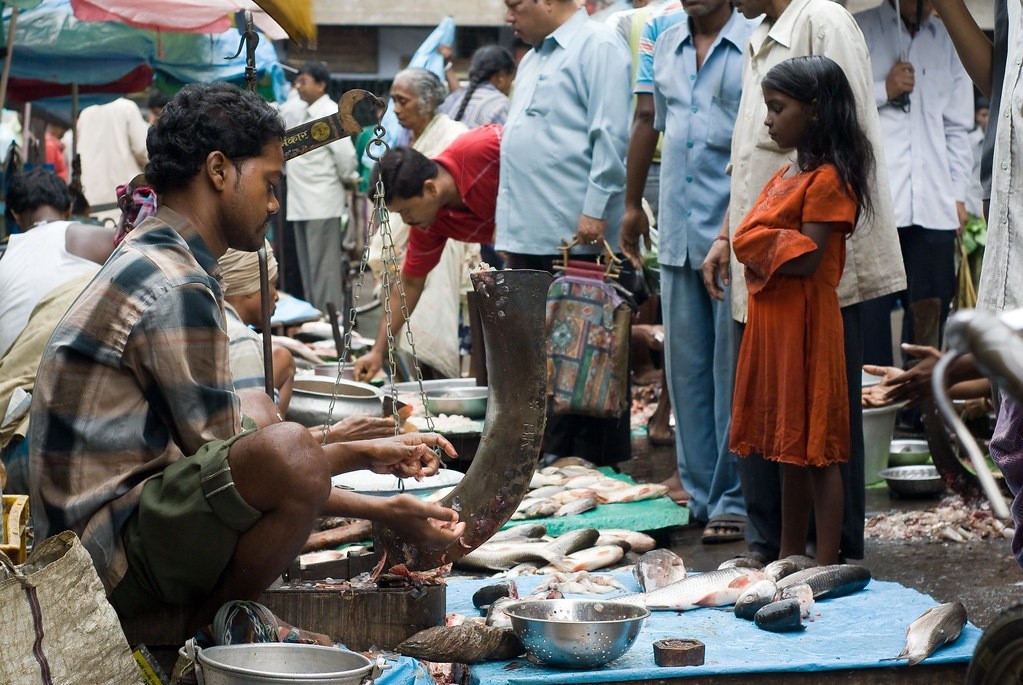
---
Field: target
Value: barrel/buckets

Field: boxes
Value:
[861,398,912,487]
[178,636,393,685]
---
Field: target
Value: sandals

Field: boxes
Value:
[700,513,749,546]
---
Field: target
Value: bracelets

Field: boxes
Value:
[714,235,730,242]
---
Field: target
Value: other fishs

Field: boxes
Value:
[512,464,668,520]
[878,600,967,667]
[463,525,871,629]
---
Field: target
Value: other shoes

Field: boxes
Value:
[733,551,769,567]
[246,611,331,645]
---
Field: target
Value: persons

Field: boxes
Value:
[389,68,482,381]
[729,55,877,566]
[494,0,634,472]
[45,92,174,228]
[861,344,1023,570]
[927,0,1023,317]
[587,0,766,542]
[281,61,359,318]
[703,0,908,566]
[438,37,533,129]
[354,123,503,384]
[969,104,990,219]
[29,82,465,645]
[0,168,116,495]
[215,237,296,415]
[849,0,975,364]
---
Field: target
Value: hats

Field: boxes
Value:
[214,239,278,296]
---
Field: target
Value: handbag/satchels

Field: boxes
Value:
[540,234,632,419]
[957,231,977,311]
[1,532,144,685]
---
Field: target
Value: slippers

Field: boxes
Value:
[646,416,675,447]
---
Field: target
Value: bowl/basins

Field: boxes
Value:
[379,376,476,395]
[878,464,946,495]
[312,362,355,380]
[503,598,651,669]
[421,386,487,417]
[287,377,384,429]
[889,438,930,464]
